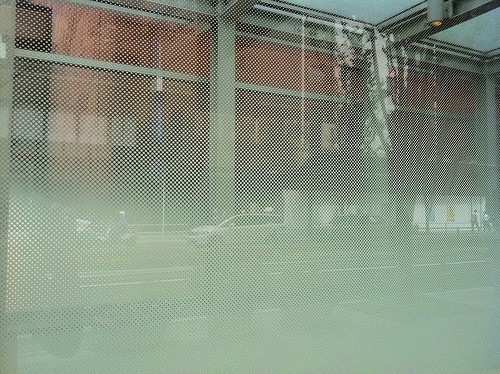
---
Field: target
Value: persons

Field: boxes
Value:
[116,211,127,232]
[482,211,492,231]
[471,209,481,231]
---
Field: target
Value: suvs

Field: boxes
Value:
[189,213,281,245]
